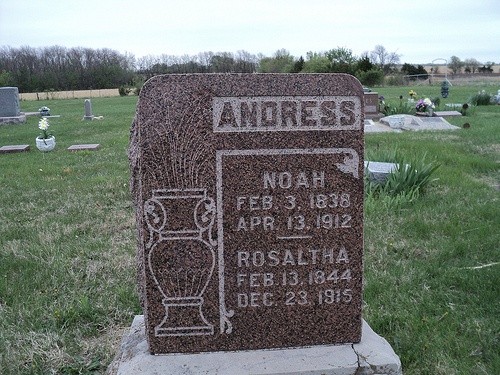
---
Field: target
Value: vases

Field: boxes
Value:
[36,136,56,152]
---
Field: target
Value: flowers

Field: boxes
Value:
[37,118,55,145]
